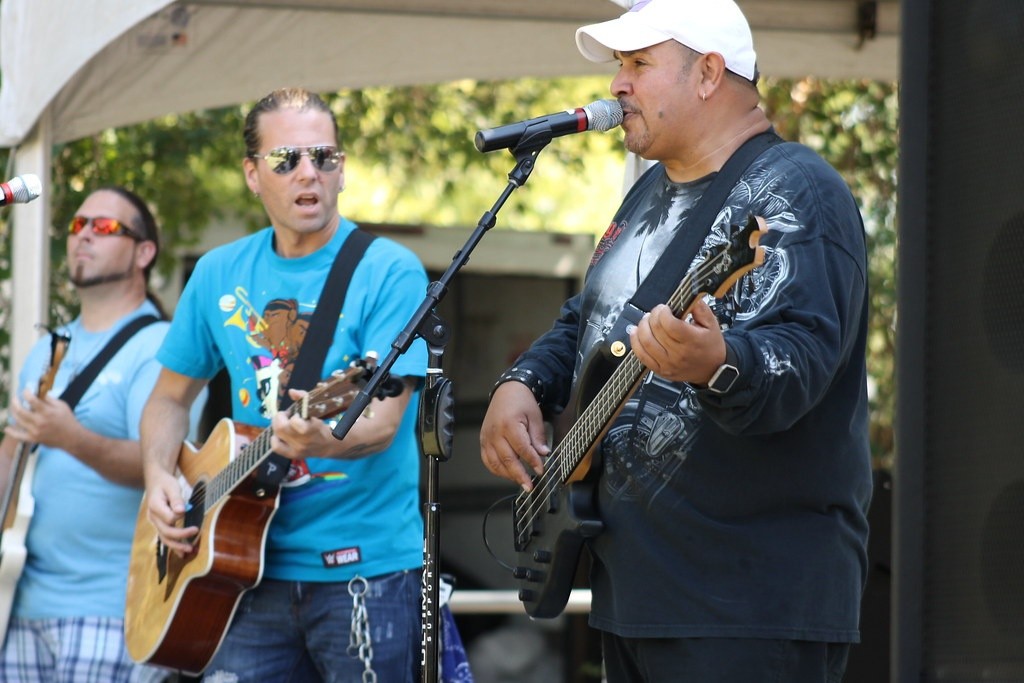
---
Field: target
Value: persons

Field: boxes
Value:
[479,0,870,683]
[139,89,430,683]
[0,187,208,683]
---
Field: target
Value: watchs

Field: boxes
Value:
[683,341,740,394]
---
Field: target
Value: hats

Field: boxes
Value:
[575,0,757,81]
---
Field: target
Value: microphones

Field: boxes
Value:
[0,174,42,209]
[474,98,624,154]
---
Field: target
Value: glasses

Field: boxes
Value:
[66,216,141,243]
[254,145,341,176]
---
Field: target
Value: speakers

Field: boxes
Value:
[887,0,1024,683]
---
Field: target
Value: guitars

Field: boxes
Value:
[0,338,66,645]
[511,216,770,619]
[121,354,378,675]
